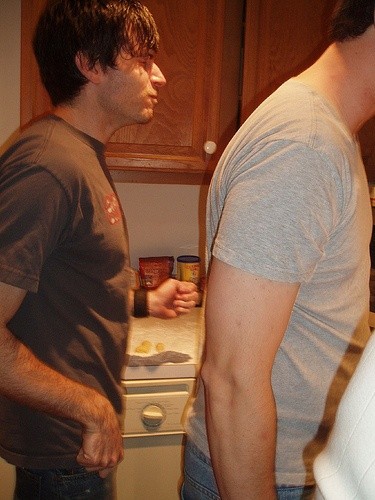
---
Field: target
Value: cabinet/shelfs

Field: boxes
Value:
[0,307,206,500]
[18,0,375,181]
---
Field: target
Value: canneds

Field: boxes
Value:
[176,255,201,287]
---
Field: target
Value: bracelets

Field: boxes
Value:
[132,289,149,320]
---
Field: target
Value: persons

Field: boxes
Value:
[0,0,202,499]
[179,0,374,499]
